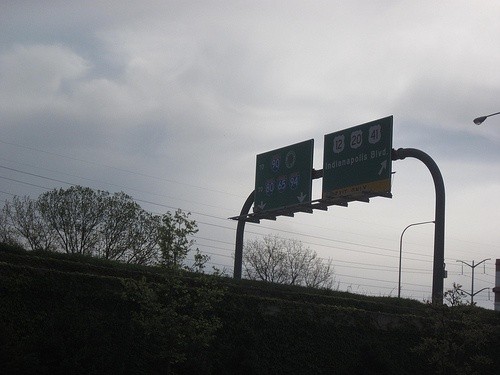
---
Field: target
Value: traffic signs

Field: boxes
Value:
[251,115,393,212]
